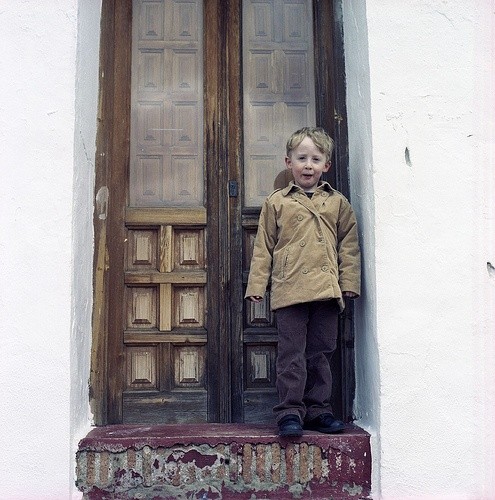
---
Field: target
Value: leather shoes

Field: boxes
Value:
[303,414,345,432]
[276,414,302,437]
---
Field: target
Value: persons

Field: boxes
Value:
[244,127,364,438]
